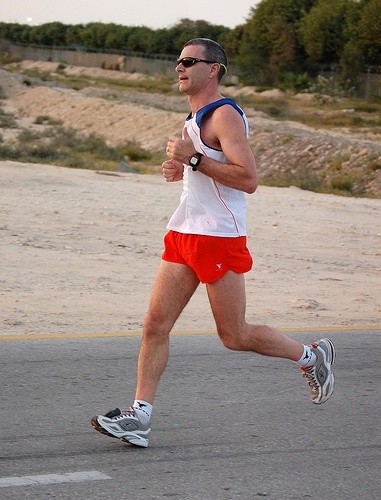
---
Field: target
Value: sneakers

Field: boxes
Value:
[299,337,337,405]
[91,406,153,449]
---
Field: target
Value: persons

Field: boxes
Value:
[90,37,336,448]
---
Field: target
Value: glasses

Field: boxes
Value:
[175,57,220,68]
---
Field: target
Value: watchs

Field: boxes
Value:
[189,152,203,171]
